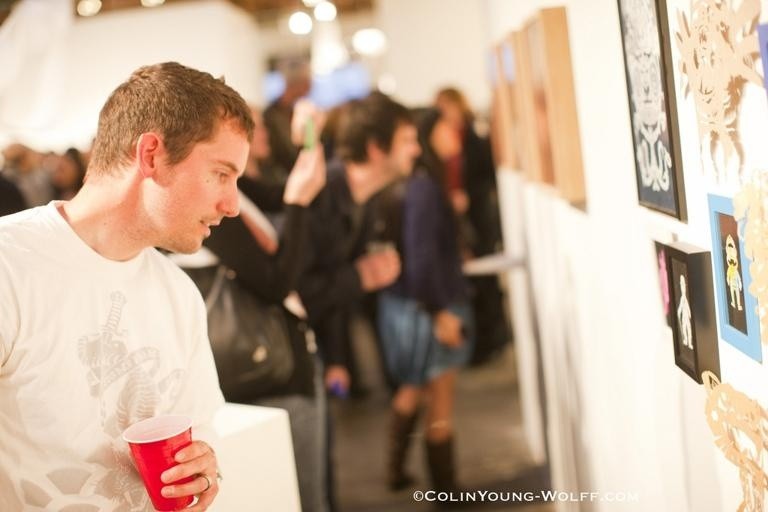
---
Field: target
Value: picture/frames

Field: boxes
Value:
[613,0,721,392]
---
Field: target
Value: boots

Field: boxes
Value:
[389,411,416,488]
[423,435,475,509]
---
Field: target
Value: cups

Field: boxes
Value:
[120,412,195,512]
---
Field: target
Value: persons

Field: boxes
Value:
[2,58,514,511]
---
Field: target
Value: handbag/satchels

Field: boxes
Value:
[199,269,294,400]
